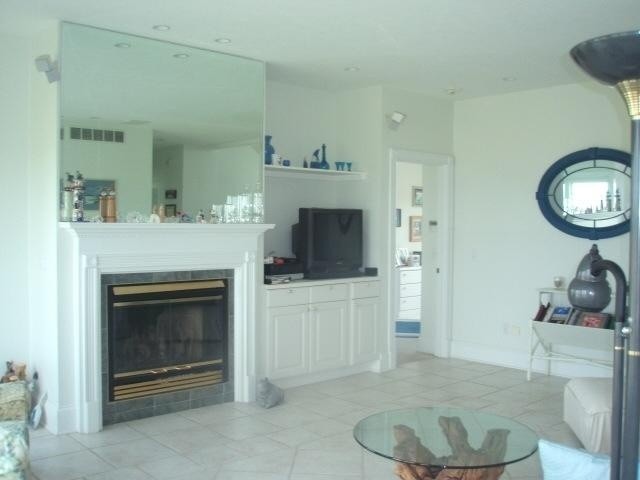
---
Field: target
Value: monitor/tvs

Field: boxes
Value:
[291,207,363,280]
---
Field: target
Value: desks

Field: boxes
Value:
[352,406,540,479]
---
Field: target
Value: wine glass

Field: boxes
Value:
[554,276,561,290]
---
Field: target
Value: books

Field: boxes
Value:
[534,302,612,331]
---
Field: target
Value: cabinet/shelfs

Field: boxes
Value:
[525,275,628,382]
[257,279,349,383]
[392,266,423,320]
[350,276,386,374]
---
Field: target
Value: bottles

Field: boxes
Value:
[320,143,330,170]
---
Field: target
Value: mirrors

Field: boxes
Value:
[537,146,636,244]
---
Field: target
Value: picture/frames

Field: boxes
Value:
[396,185,424,242]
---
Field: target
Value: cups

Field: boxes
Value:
[334,161,352,172]
[271,154,282,166]
